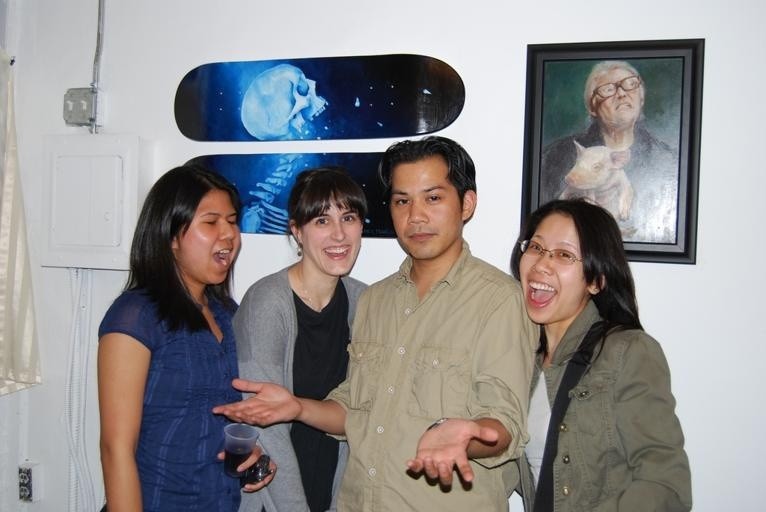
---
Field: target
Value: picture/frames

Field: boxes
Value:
[521,38,704,268]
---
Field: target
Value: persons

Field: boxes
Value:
[226,164,370,512]
[538,60,679,244]
[212,136,541,512]
[509,197,692,511]
[97,164,277,511]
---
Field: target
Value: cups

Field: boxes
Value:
[223,423,260,479]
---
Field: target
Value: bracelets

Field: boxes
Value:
[425,418,448,431]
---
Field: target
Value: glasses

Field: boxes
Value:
[590,76,641,113]
[516,240,583,265]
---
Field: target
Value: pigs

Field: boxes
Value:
[558,140,634,224]
